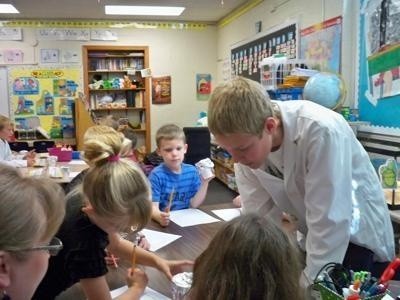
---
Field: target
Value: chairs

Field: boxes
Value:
[8,140,55,153]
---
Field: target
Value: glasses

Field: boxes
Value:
[7,236,64,257]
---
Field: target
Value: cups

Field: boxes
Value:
[194,157,215,181]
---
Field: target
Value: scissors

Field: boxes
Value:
[367,258,400,295]
[353,271,370,283]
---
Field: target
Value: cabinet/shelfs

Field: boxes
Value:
[81,44,152,156]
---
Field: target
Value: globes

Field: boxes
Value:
[302,70,347,111]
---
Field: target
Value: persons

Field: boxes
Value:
[25,125,195,299]
[185,210,302,299]
[0,113,38,168]
[0,163,67,299]
[204,77,397,294]
[145,123,209,226]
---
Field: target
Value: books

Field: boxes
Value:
[88,56,146,108]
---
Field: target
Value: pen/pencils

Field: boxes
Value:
[132,245,137,272]
[165,192,173,214]
[323,263,388,300]
